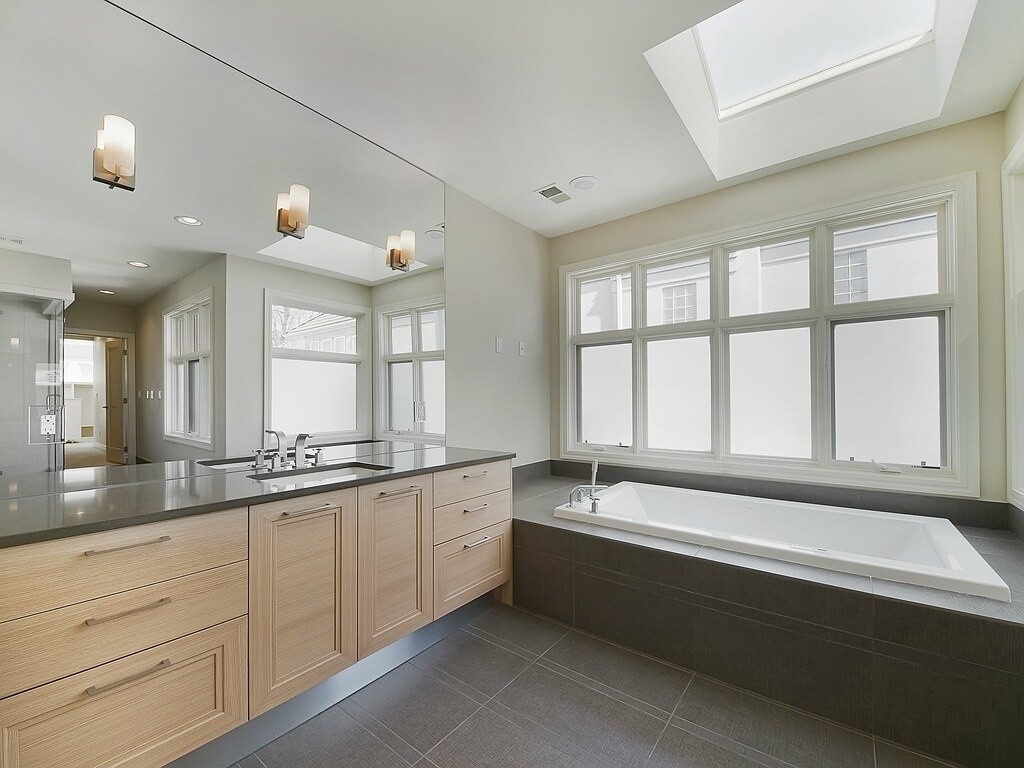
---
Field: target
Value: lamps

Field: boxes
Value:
[385,234,400,271]
[276,192,290,238]
[92,114,138,192]
[95,129,115,190]
[392,229,416,272]
[278,183,311,239]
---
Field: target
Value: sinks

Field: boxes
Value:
[196,452,316,470]
[243,461,394,485]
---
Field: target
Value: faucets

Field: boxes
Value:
[569,485,610,507]
[265,429,287,462]
[294,432,313,468]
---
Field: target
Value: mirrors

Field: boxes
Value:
[0,1,446,499]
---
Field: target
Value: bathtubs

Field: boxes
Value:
[554,481,1011,767]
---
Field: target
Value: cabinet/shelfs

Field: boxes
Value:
[434,458,513,621]
[64,397,83,442]
[0,505,249,768]
[246,487,357,719]
[358,471,434,663]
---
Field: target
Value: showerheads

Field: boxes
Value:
[590,459,598,496]
